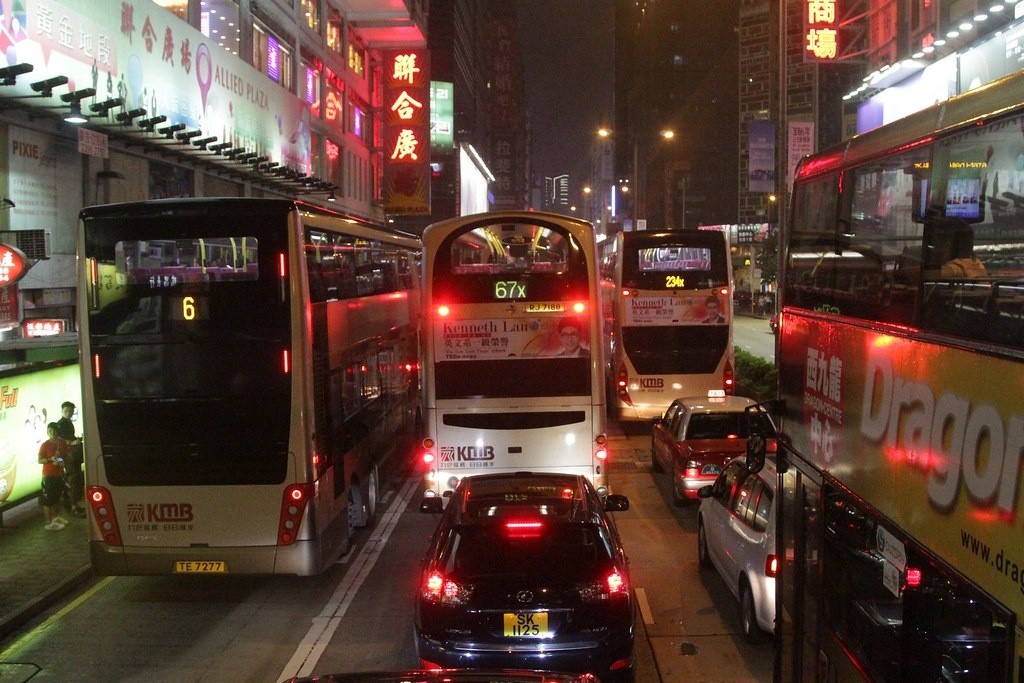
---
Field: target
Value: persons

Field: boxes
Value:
[701,295,725,324]
[161,246,175,264]
[37,422,69,531]
[504,244,510,253]
[927,219,991,299]
[556,316,590,356]
[57,401,87,519]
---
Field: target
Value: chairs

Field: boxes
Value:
[459,262,557,274]
[640,260,708,270]
[125,263,390,304]
[786,244,1024,353]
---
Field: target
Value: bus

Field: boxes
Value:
[593,229,737,424]
[76,194,440,579]
[743,68,1023,683]
[413,210,606,509]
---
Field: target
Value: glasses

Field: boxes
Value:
[707,306,718,312]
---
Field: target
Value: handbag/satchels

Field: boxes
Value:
[67,438,83,464]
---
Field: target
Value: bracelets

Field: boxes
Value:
[48,458,54,463]
[69,440,72,446]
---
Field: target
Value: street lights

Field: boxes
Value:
[594,126,677,231]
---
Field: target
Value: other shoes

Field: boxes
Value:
[71,506,86,519]
[52,515,68,525]
[61,493,73,506]
[45,520,65,530]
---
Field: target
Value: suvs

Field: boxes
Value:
[698,451,780,644]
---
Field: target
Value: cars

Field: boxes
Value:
[651,389,781,508]
[411,470,639,682]
